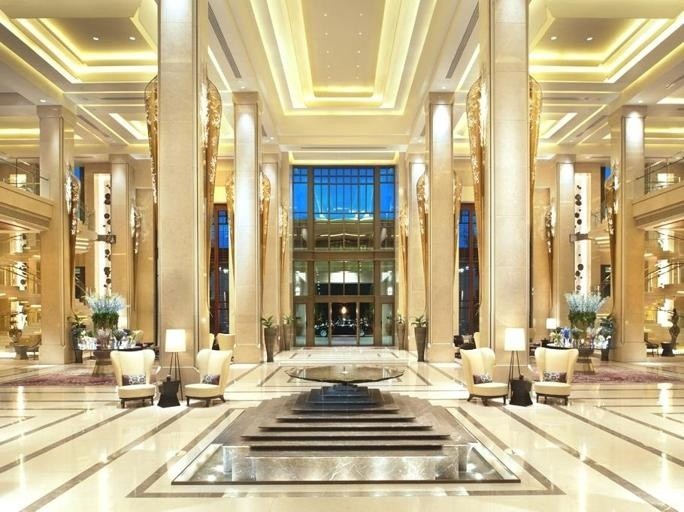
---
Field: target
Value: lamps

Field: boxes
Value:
[164,328,187,400]
[504,327,526,381]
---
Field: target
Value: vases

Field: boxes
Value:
[95,325,111,350]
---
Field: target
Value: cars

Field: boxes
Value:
[315,319,368,337]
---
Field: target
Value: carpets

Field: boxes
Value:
[1,374,117,386]
[529,362,683,384]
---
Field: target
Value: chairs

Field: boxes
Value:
[110,348,155,408]
[459,347,508,406]
[534,346,579,405]
[183,348,232,407]
[209,333,235,357]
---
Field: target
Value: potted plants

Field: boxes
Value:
[396,314,406,350]
[66,310,88,363]
[411,314,429,362]
[281,313,294,350]
[261,315,275,362]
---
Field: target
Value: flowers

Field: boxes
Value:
[548,290,611,351]
[80,287,130,334]
